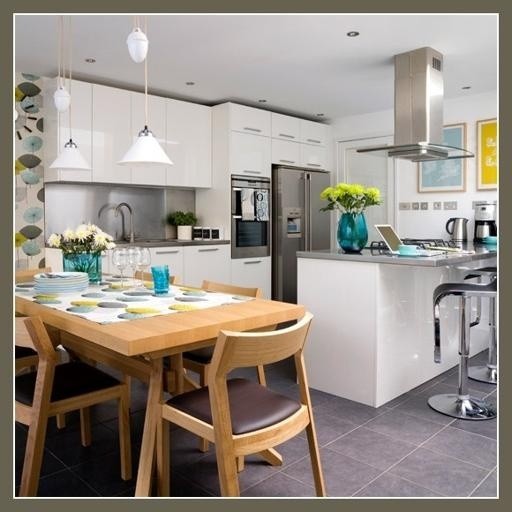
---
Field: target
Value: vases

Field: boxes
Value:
[337,214,368,254]
[62,253,102,285]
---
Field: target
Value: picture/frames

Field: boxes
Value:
[476,117,500,191]
[417,123,466,192]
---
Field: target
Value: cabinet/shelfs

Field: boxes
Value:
[229,258,273,300]
[232,102,334,174]
[44,244,229,287]
[38,75,214,190]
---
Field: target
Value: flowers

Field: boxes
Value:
[318,183,385,212]
[48,221,117,253]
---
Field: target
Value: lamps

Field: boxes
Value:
[48,15,93,171]
[117,15,174,173]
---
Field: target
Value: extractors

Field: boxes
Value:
[355,46,476,163]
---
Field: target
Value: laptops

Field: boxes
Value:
[374,224,446,257]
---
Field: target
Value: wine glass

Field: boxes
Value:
[112,245,151,290]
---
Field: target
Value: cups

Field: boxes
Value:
[397,244,421,256]
[151,264,171,295]
[193,227,221,242]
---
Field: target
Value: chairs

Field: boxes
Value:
[427,277,497,421]
[16,266,52,283]
[162,311,329,497]
[15,314,132,497]
[163,279,267,453]
[134,269,177,293]
[15,345,65,428]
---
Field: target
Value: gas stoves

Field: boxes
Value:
[364,236,462,252]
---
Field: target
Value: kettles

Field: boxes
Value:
[445,217,470,242]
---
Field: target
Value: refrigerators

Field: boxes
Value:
[271,163,333,330]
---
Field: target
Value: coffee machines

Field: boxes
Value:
[471,200,497,246]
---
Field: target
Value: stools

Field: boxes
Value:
[463,265,498,385]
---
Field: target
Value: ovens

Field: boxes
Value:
[230,174,273,261]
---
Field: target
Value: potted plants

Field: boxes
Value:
[168,208,199,241]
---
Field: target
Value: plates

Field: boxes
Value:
[34,270,90,296]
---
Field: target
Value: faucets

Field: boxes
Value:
[115,203,134,236]
